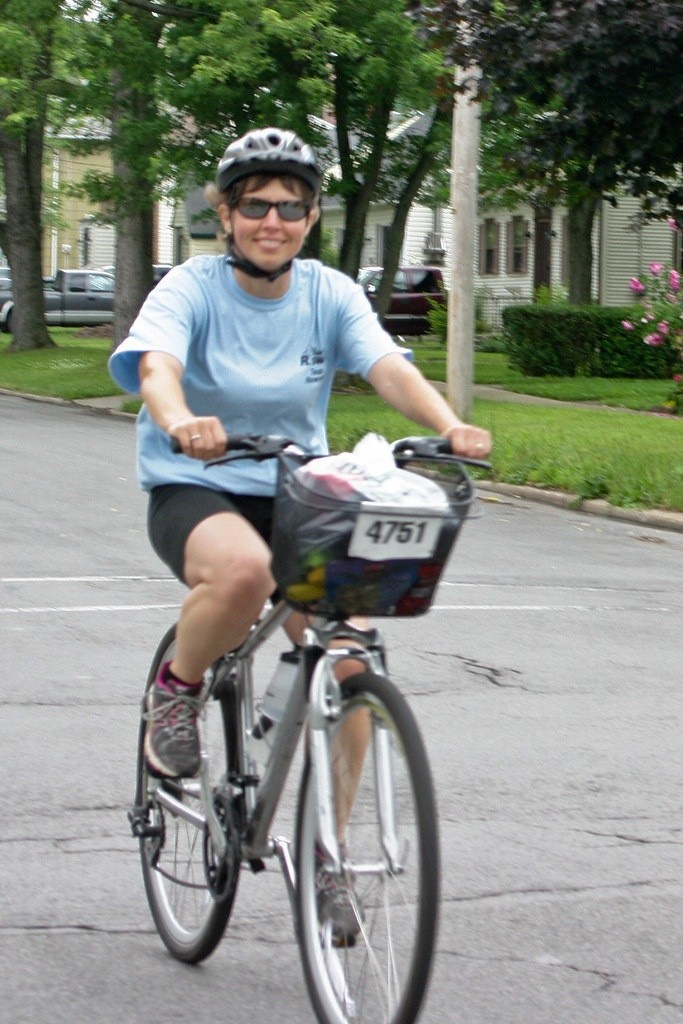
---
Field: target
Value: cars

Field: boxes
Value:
[354,262,445,332]
[105,263,172,291]
[357,266,383,291]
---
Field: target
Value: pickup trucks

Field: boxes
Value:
[0,269,118,334]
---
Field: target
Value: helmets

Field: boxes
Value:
[217,125,324,194]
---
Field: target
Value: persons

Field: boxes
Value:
[106,126,493,947]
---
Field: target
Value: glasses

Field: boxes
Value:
[230,198,309,220]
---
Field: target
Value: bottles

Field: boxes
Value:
[263,641,305,728]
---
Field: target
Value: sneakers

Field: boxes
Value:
[309,841,365,949]
[141,659,203,779]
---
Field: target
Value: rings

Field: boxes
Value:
[189,434,200,440]
[476,443,485,449]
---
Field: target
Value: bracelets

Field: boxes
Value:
[440,424,459,436]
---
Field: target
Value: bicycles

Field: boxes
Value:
[126,426,493,1024]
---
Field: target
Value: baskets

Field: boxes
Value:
[270,451,474,616]
